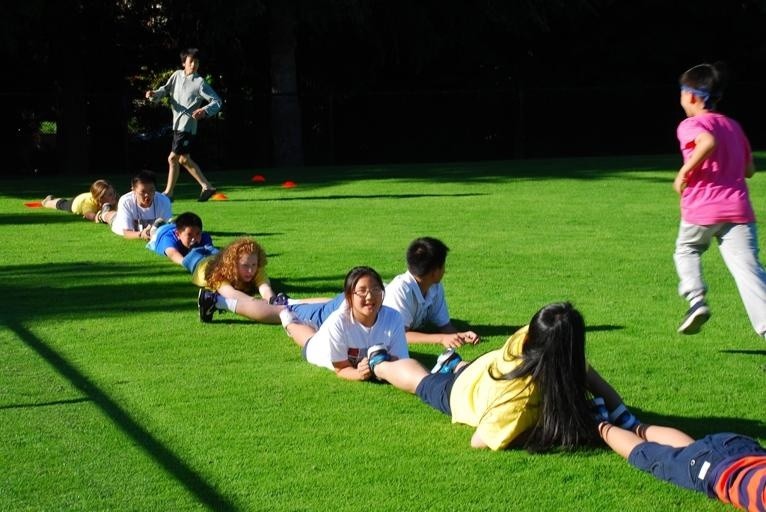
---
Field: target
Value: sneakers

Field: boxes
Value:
[431,348,462,374]
[198,187,216,201]
[197,288,217,322]
[101,203,111,223]
[367,345,388,371]
[678,299,710,334]
[587,397,609,422]
[610,402,639,429]
[273,292,288,304]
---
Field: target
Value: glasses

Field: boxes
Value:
[352,287,382,297]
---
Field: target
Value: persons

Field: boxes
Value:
[277,265,411,383]
[41,180,118,220]
[144,47,222,202]
[368,301,643,453]
[144,210,213,267]
[92,170,174,243]
[196,235,480,350]
[182,238,278,305]
[671,63,766,345]
[583,394,766,512]
